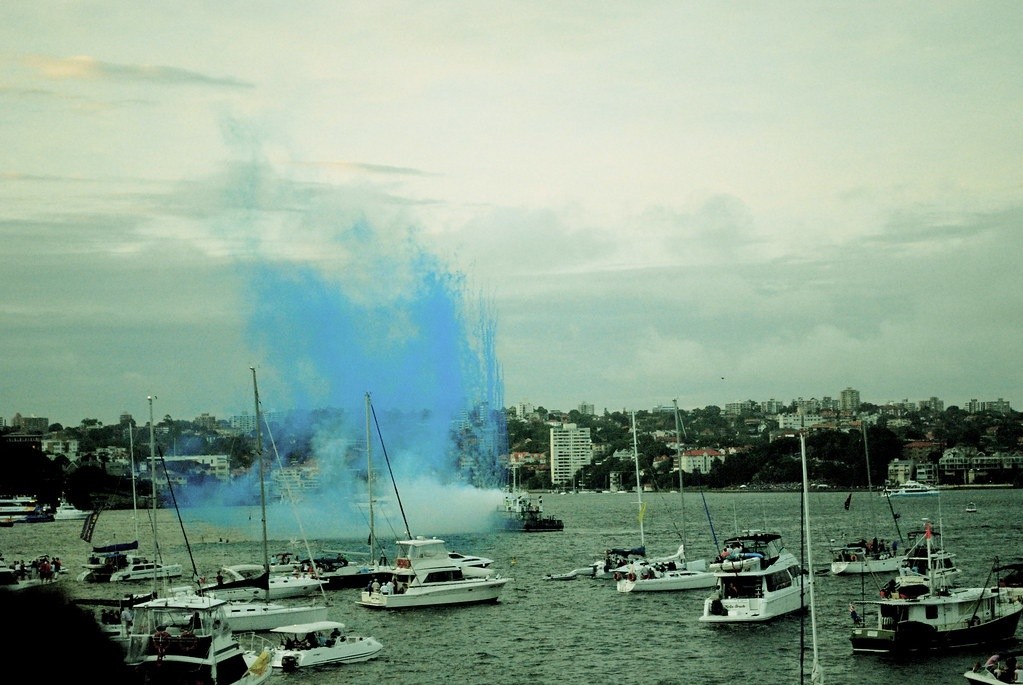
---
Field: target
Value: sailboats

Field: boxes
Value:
[72,365,331,634]
[614,399,721,592]
[309,392,496,591]
[829,425,907,574]
[589,410,686,580]
[78,420,183,585]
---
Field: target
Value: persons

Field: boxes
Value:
[714,583,737,597]
[9,555,62,584]
[88,552,128,571]
[285,628,341,650]
[984,651,1017,684]
[378,545,387,566]
[217,572,224,585]
[504,494,543,512]
[719,542,743,557]
[865,536,897,559]
[189,612,202,629]
[364,574,408,595]
[269,552,345,575]
[602,553,677,579]
[97,607,133,639]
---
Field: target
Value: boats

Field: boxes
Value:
[699,502,810,624]
[354,536,508,610]
[487,467,564,533]
[347,492,396,522]
[965,502,977,513]
[0,507,55,527]
[199,562,331,603]
[53,493,96,520]
[896,517,962,588]
[849,494,1023,656]
[126,593,275,685]
[269,620,384,671]
[963,664,1023,685]
[270,552,303,573]
[24,563,68,575]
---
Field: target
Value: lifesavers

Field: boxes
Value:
[179,632,197,651]
[850,554,857,560]
[628,572,635,581]
[276,552,293,564]
[153,631,172,652]
[613,572,621,581]
